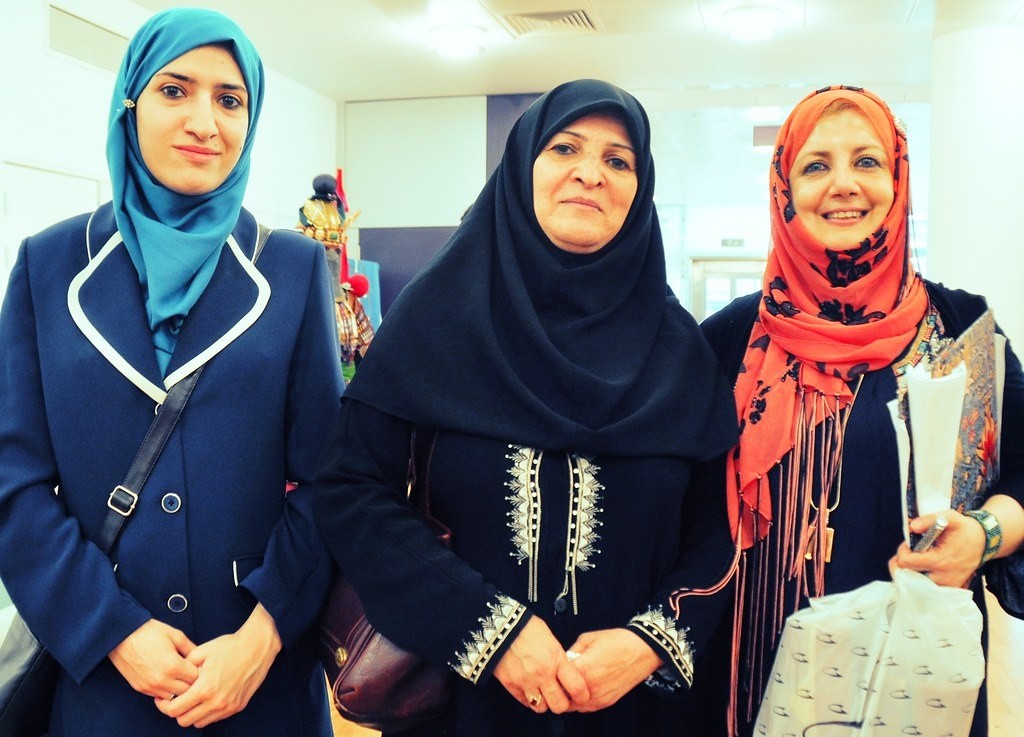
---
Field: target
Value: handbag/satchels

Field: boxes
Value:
[317,498,451,733]
[0,610,59,737]
[753,568,985,737]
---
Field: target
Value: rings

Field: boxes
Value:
[169,693,178,701]
[529,693,542,707]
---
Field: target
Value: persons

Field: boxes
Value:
[698,84,1024,737]
[310,78,741,737]
[0,8,347,737]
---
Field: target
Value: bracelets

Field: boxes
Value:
[964,509,1003,570]
